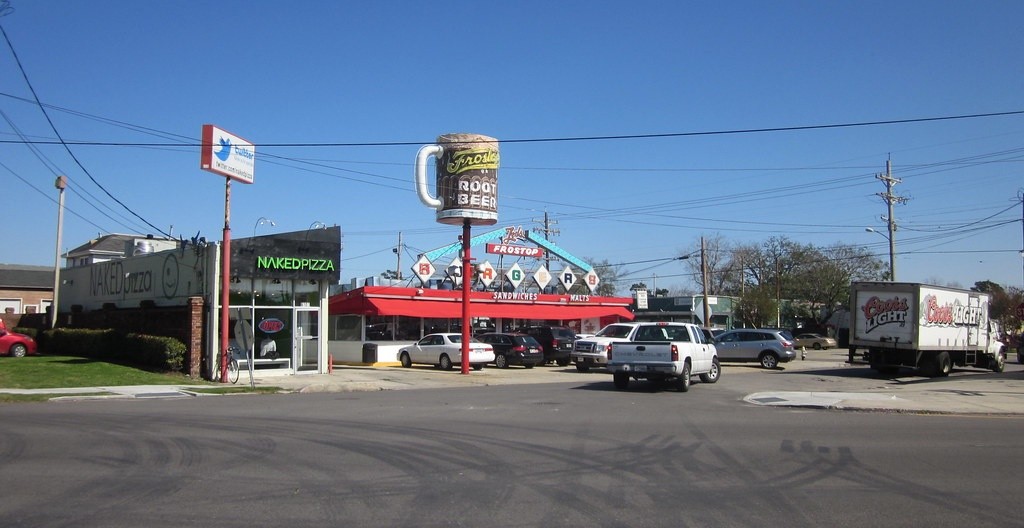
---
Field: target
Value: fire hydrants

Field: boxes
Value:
[800,345,807,360]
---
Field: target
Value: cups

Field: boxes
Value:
[415,134,500,225]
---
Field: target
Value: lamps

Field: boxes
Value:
[126,270,150,278]
[63,279,73,286]
[560,297,567,301]
[236,277,241,284]
[277,278,282,285]
[308,279,317,285]
[252,217,277,243]
[417,288,424,294]
[306,219,328,242]
[230,276,235,283]
[272,278,276,285]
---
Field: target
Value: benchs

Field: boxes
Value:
[236,358,291,372]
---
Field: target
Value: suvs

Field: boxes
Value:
[701,327,796,369]
[512,324,578,367]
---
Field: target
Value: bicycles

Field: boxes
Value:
[217,347,240,384]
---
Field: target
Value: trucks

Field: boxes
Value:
[849,280,1009,377]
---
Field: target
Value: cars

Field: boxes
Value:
[0,318,38,358]
[793,333,836,350]
[473,332,544,369]
[397,333,496,371]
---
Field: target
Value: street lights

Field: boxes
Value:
[866,227,895,281]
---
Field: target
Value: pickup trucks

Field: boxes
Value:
[570,323,637,372]
[607,321,721,392]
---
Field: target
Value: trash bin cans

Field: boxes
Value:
[362,343,378,363]
